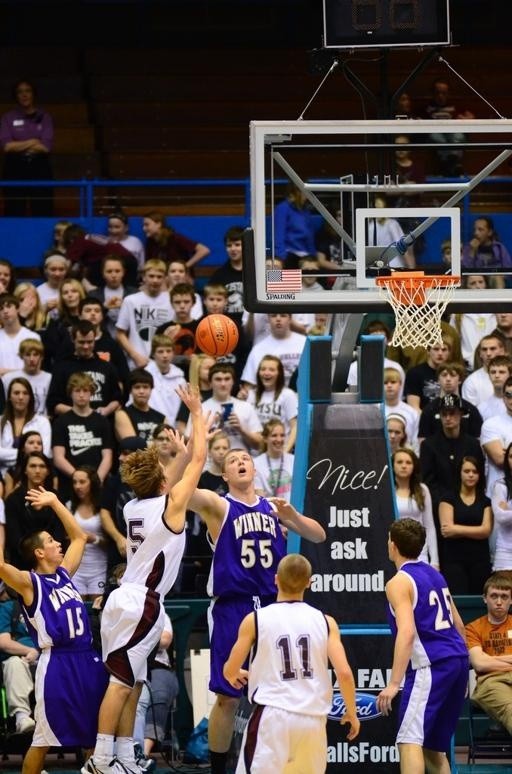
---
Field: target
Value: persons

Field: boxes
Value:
[449,275,498,372]
[175,352,218,437]
[464,575,512,738]
[187,431,232,560]
[242,256,283,346]
[0,584,42,733]
[490,441,512,582]
[48,319,122,420]
[438,456,493,593]
[384,367,419,448]
[43,279,86,371]
[461,336,510,407]
[113,369,168,450]
[418,361,483,440]
[238,310,308,399]
[479,376,512,507]
[252,416,294,505]
[237,354,299,457]
[52,221,71,256]
[462,215,511,289]
[366,196,415,271]
[113,259,174,370]
[404,331,453,414]
[14,283,47,335]
[53,371,115,514]
[474,313,512,371]
[0,291,41,375]
[86,253,141,340]
[79,383,222,774]
[346,321,406,404]
[419,393,485,503]
[36,254,67,321]
[74,298,114,361]
[149,424,176,473]
[477,355,512,422]
[392,448,440,573]
[422,82,474,177]
[388,137,427,255]
[62,466,129,610]
[150,283,201,357]
[142,212,210,280]
[4,431,58,500]
[271,175,322,257]
[143,335,184,429]
[388,92,424,136]
[1,338,52,416]
[313,312,326,333]
[375,517,469,774]
[315,203,354,288]
[166,259,203,320]
[0,484,107,774]
[3,451,61,564]
[0,257,13,296]
[385,412,407,451]
[61,224,138,294]
[222,554,360,774]
[0,377,52,468]
[183,362,262,471]
[106,207,145,281]
[209,225,247,325]
[293,258,324,330]
[197,284,243,358]
[440,240,463,273]
[166,408,325,774]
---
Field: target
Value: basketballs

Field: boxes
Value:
[194,314,238,354]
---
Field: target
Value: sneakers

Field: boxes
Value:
[81,757,135,774]
[136,753,155,770]
[14,716,35,734]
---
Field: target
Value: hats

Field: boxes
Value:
[440,393,464,418]
